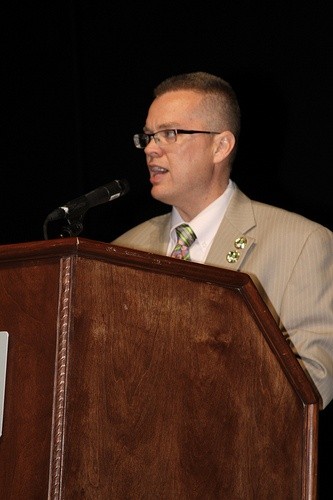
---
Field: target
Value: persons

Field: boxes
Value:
[109,70,332,413]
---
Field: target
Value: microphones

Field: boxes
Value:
[47,178,129,222]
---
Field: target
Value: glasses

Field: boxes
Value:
[134,129,223,148]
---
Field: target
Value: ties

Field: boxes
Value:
[171,225,197,260]
